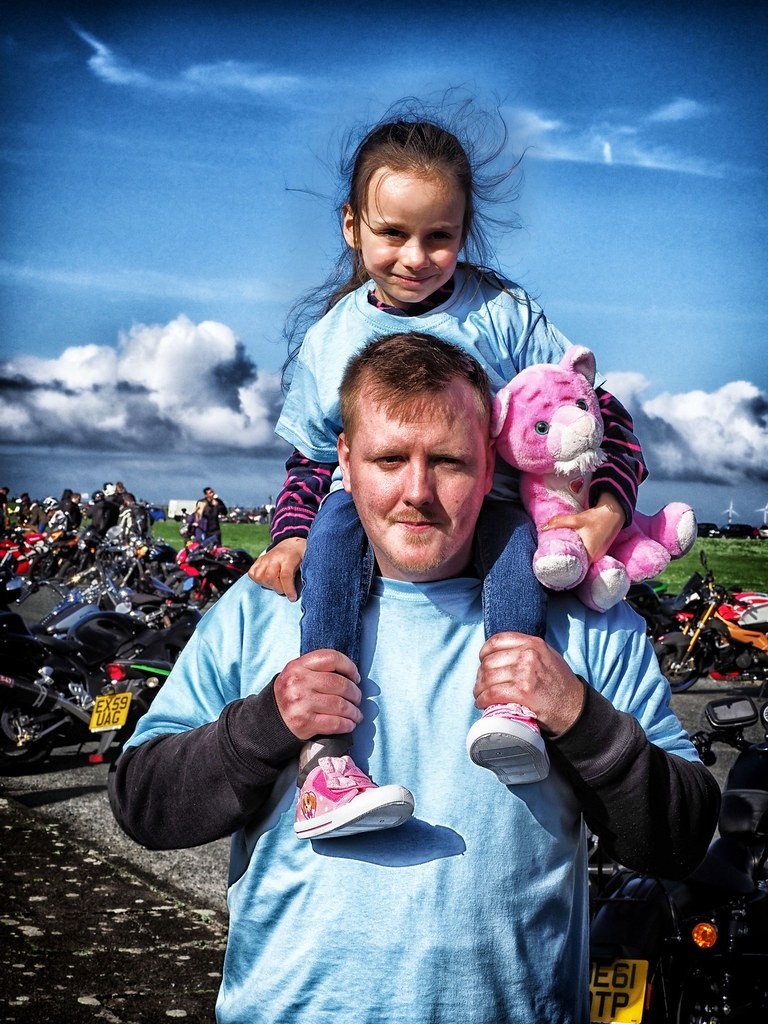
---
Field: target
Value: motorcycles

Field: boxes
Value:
[0,525,257,607]
[648,549,768,696]
[1,570,203,765]
[0,526,259,606]
[625,579,679,638]
[588,696,768,1024]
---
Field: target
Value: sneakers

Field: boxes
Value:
[293,755,415,840]
[466,703,550,786]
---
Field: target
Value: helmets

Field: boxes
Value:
[42,496,60,515]
[92,491,105,504]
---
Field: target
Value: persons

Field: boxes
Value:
[249,120,649,843]
[106,332,726,1024]
[0,482,152,534]
[196,487,229,547]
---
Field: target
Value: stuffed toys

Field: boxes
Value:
[488,341,699,616]
